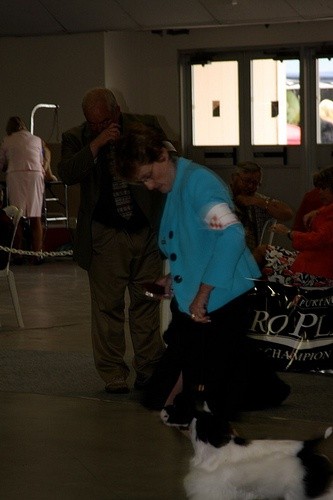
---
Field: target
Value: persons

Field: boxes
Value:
[114,121,262,428]
[58,87,172,394]
[231,160,293,262]
[0,116,58,266]
[267,165,333,279]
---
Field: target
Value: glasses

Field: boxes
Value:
[238,174,262,188]
[83,109,114,129]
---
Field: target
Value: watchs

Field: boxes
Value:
[264,196,273,208]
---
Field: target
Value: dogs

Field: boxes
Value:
[157,380,333,500]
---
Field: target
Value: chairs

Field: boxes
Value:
[0,207,25,328]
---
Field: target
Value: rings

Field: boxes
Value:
[192,314,195,318]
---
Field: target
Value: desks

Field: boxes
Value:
[40,182,72,243]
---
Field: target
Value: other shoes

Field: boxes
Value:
[29,256,42,264]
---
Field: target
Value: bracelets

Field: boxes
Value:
[287,229,292,240]
